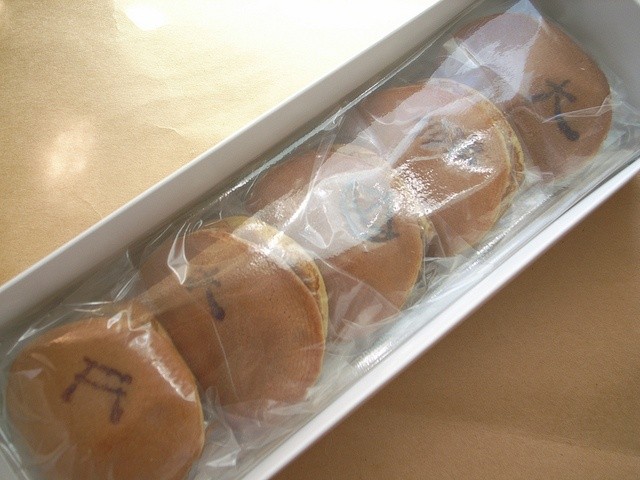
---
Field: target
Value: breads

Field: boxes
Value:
[249,143,423,346]
[6,305,206,479]
[359,79,525,258]
[435,12,612,187]
[140,216,329,426]
